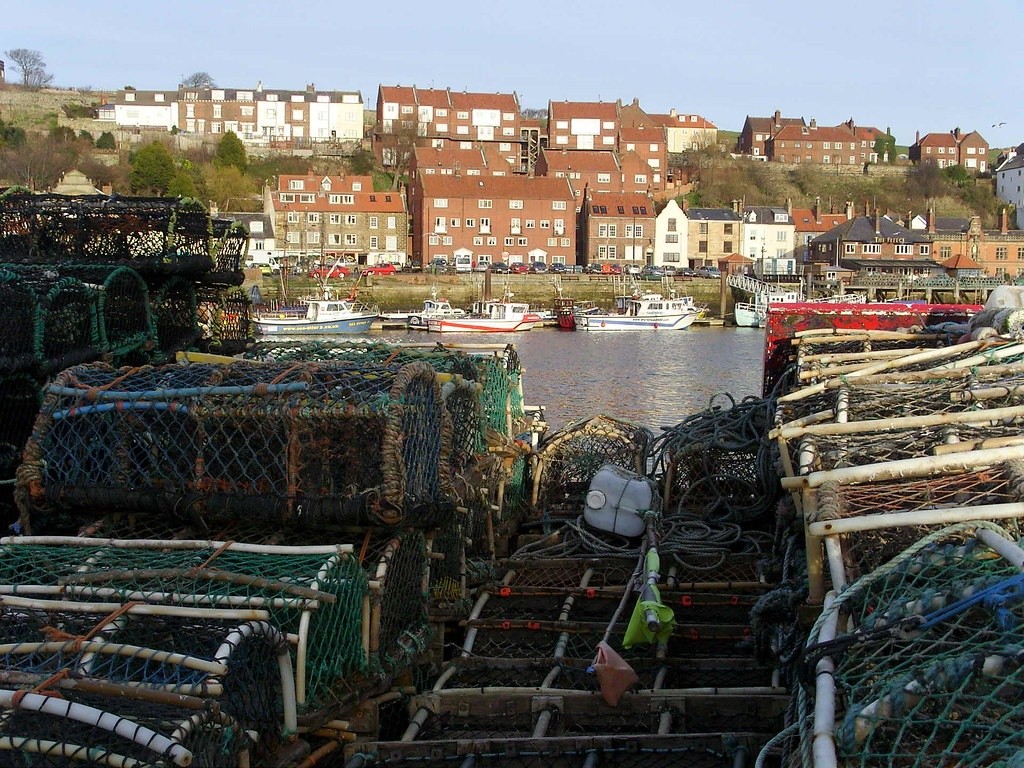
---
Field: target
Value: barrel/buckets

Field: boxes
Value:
[582,466,651,536]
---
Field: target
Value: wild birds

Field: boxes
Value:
[992,122,1006,127]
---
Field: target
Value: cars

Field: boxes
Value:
[245,255,721,278]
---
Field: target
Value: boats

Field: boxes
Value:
[427,297,542,332]
[529,299,608,325]
[377,282,472,331]
[727,273,868,328]
[572,296,704,332]
[250,290,381,336]
